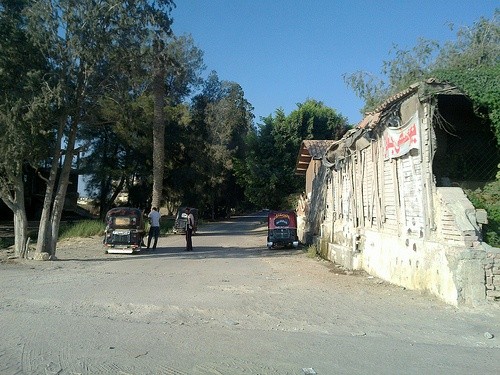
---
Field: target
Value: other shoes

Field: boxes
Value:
[153,246,156,249]
[184,249,189,251]
[147,246,149,249]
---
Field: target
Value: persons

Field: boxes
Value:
[184,208,194,251]
[145,207,161,251]
